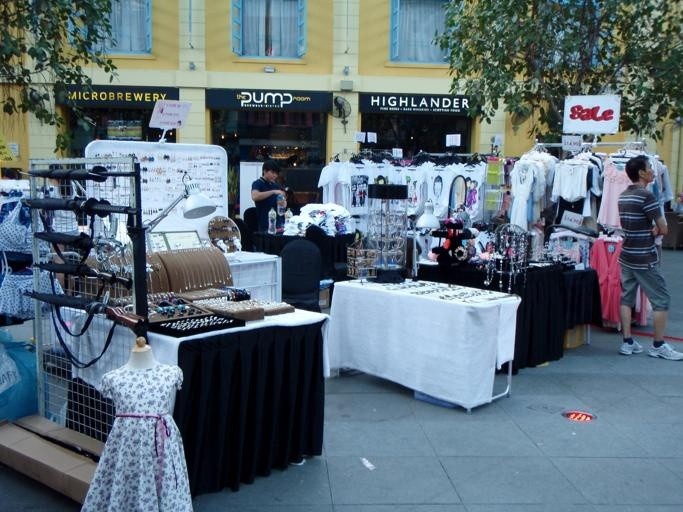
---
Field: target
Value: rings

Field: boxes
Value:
[159,316,234,331]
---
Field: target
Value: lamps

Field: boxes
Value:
[143,164,216,247]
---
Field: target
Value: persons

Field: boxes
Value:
[617,153,683,361]
[251,159,288,231]
[78,335,194,512]
[351,189,367,207]
[465,176,478,210]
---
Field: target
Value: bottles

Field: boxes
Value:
[284,208,293,224]
[276,193,285,217]
[267,206,279,236]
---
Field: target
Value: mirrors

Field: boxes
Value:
[446,175,467,219]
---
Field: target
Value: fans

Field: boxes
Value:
[326,93,350,135]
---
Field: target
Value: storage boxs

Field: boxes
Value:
[0,413,104,506]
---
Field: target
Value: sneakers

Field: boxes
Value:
[649,344,683,361]
[620,340,643,355]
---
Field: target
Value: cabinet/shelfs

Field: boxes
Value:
[19,163,142,326]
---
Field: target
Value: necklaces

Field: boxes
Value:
[174,247,232,293]
[483,230,529,295]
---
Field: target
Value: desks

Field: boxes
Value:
[323,277,522,414]
[73,292,325,490]
[72,209,599,374]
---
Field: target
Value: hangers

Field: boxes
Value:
[329,139,662,168]
